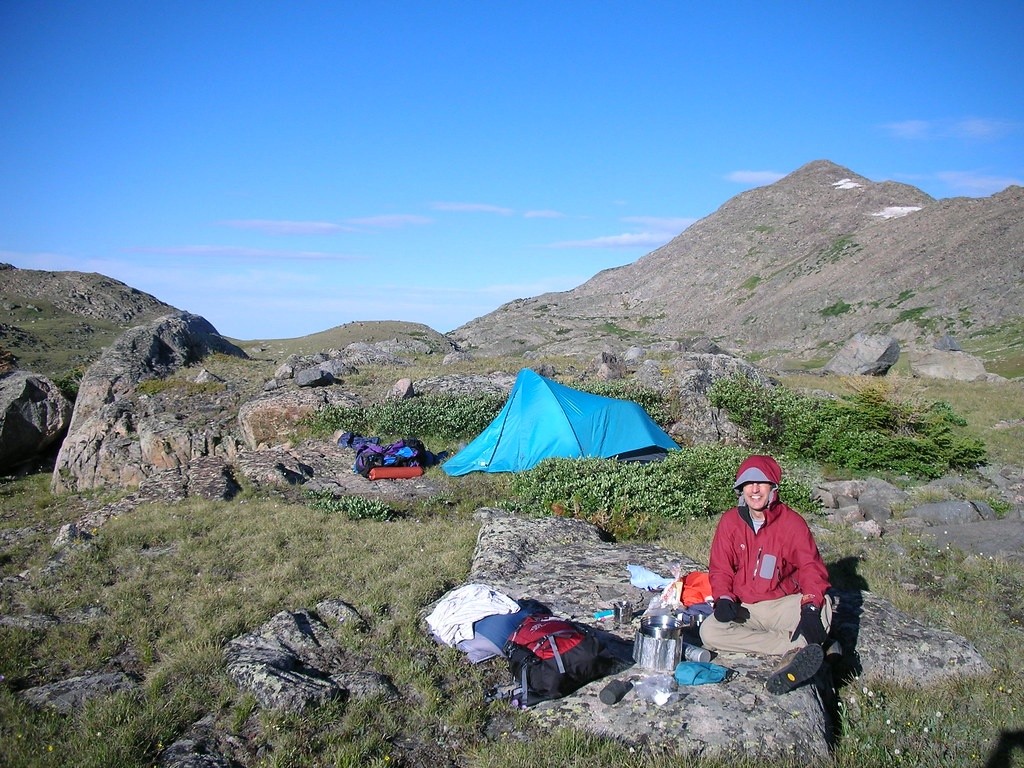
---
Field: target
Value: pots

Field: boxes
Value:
[640,615,683,637]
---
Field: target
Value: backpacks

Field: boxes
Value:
[504,612,614,708]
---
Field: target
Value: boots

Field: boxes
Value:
[766,644,823,695]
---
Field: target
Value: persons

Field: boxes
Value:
[700,454,833,699]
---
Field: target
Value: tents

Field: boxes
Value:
[441,367,687,478]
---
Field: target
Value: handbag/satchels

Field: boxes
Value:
[354,438,427,477]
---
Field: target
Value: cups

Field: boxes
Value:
[690,614,706,635]
[599,679,626,705]
[614,601,633,624]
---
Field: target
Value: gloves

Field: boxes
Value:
[714,599,744,622]
[790,604,827,645]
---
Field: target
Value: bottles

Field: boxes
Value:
[593,609,616,620]
[682,642,712,662]
